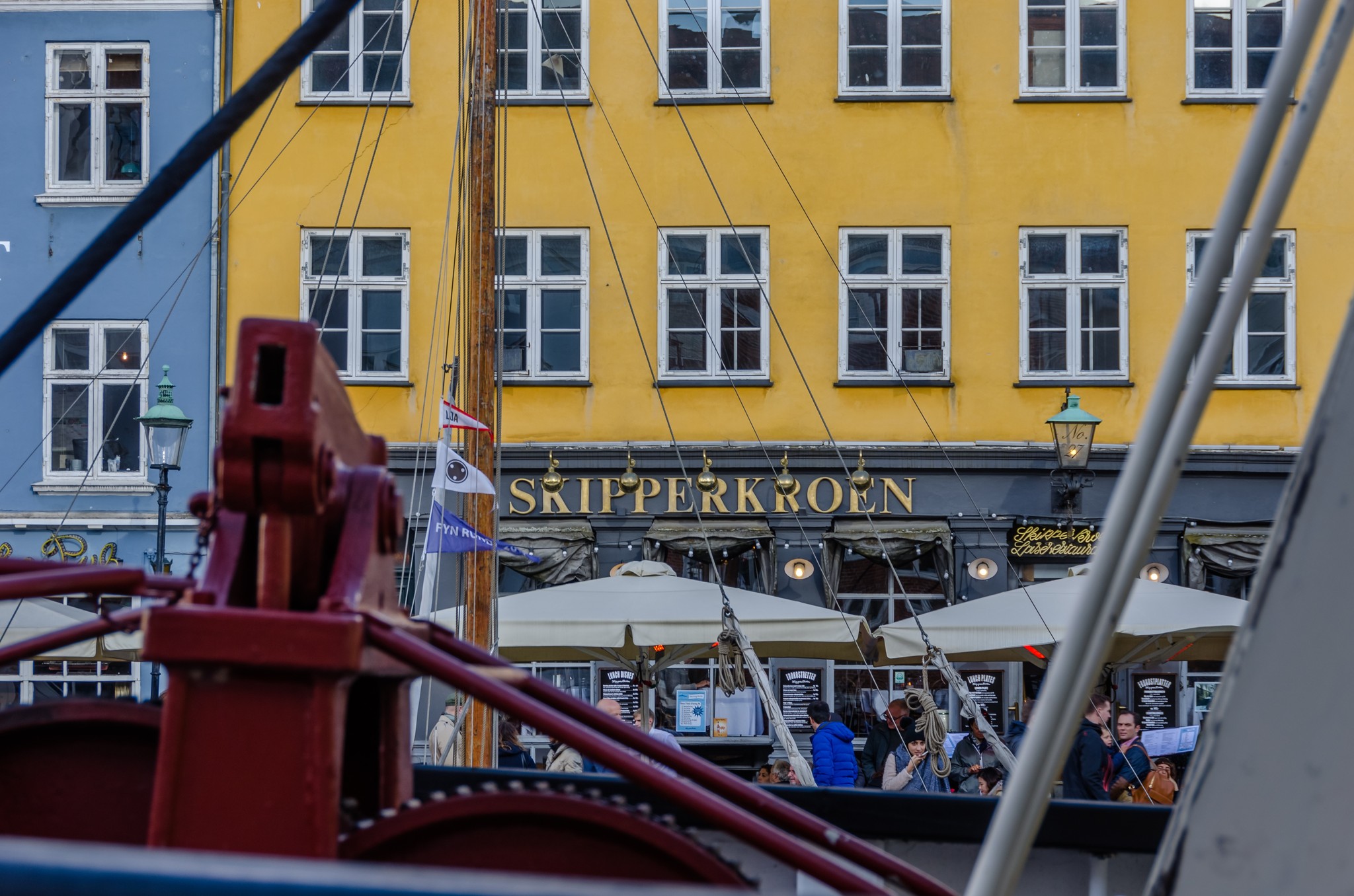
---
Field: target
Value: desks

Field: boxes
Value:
[858,688,905,722]
[930,689,949,710]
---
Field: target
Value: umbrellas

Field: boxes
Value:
[0,596,145,663]
[409,560,881,766]
[873,562,1249,743]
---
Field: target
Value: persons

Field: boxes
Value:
[429,693,1178,808]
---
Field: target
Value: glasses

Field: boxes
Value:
[883,714,905,722]
[789,770,795,775]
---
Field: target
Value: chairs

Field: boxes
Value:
[655,687,676,728]
[844,677,876,731]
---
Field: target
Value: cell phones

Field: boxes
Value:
[979,768,985,770]
[920,751,928,757]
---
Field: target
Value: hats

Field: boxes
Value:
[900,717,925,744]
[445,693,462,705]
[807,700,830,715]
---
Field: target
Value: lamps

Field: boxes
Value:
[785,557,814,580]
[1139,562,1169,583]
[653,645,665,652]
[1022,563,1035,581]
[849,448,872,492]
[618,451,641,494]
[541,450,564,493]
[1045,387,1103,525]
[610,563,626,577]
[774,451,796,495]
[696,449,718,493]
[968,558,998,580]
[149,557,173,576]
[121,108,141,176]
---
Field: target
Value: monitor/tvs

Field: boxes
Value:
[72,438,121,472]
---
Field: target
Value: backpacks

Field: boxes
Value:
[1125,744,1174,805]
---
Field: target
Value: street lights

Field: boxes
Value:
[131,366,195,706]
